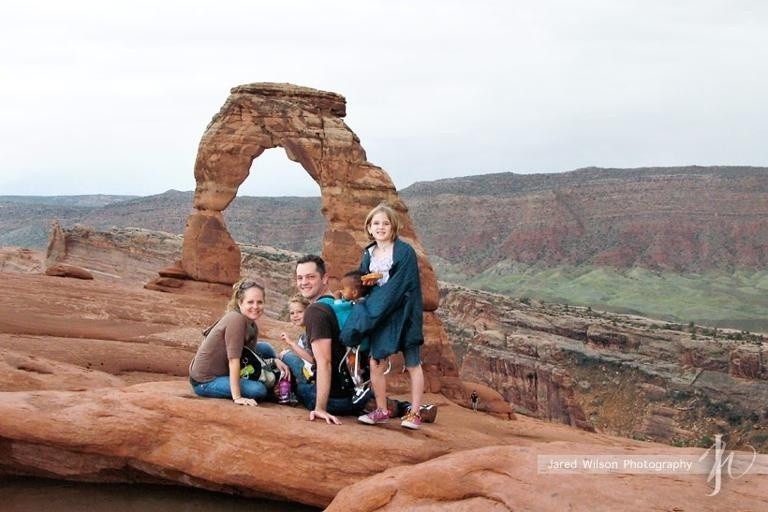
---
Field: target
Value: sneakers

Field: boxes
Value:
[401,411,422,430]
[402,403,437,423]
[358,407,389,424]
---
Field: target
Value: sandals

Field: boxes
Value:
[351,384,371,405]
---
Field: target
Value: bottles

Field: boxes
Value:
[278,377,290,403]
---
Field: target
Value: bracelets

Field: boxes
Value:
[231,395,243,403]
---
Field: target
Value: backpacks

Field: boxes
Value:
[239,345,280,390]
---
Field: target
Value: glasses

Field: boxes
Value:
[241,280,264,289]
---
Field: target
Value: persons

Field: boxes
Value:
[470,390,479,411]
[330,269,372,409]
[334,201,431,431]
[280,290,320,382]
[278,251,440,429]
[185,274,294,411]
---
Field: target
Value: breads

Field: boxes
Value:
[360,273,383,280]
